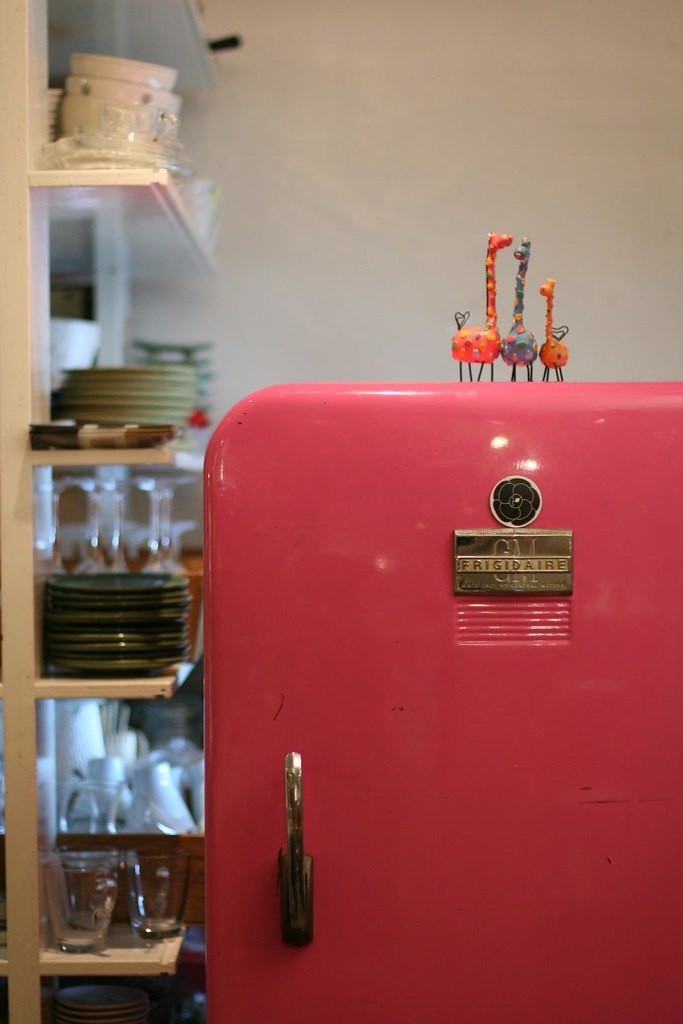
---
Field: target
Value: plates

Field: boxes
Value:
[29,363,199,448]
[54,984,150,1024]
[46,86,64,142]
[43,572,194,672]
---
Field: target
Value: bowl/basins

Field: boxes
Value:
[61,52,185,145]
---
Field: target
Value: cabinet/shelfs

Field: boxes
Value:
[0,0,227,1023]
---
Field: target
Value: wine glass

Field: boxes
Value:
[56,473,196,575]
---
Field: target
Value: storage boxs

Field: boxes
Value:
[58,833,205,926]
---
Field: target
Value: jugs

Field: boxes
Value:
[60,740,202,835]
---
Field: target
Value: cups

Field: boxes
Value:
[45,860,117,953]
[49,315,103,391]
[55,841,124,930]
[125,846,191,939]
